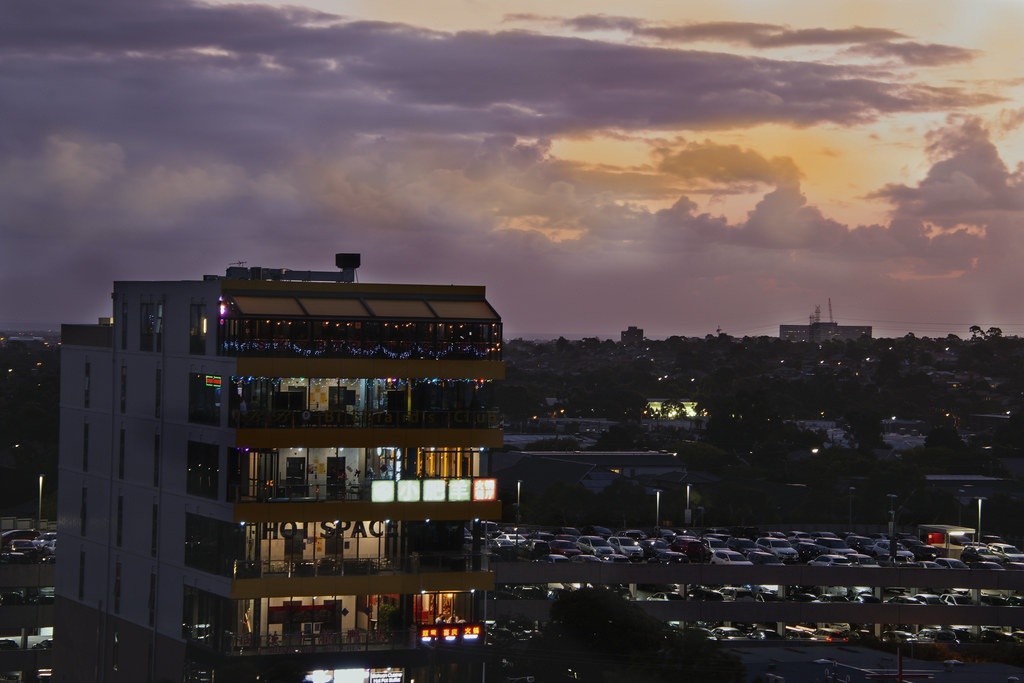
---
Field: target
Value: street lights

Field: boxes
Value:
[957,489,964,527]
[849,486,856,526]
[686,484,692,509]
[515,479,525,558]
[974,496,989,547]
[886,494,898,537]
[655,489,664,539]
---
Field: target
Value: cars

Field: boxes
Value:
[0,587,56,605]
[463,519,1024,570]
[935,558,971,569]
[576,537,615,554]
[0,639,19,649]
[0,530,58,556]
[30,639,53,650]
[871,540,915,561]
[987,544,1024,562]
[846,536,877,553]
[806,554,853,566]
[847,553,882,568]
[487,622,1023,645]
[972,561,1005,570]
[709,550,754,565]
[607,537,644,559]
[489,581,1023,617]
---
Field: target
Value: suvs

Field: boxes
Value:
[813,537,858,556]
[755,538,801,562]
